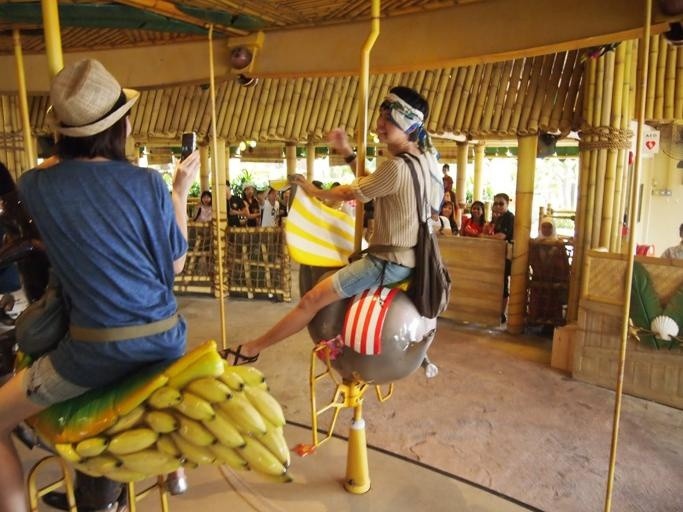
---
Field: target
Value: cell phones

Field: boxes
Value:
[180,131,197,164]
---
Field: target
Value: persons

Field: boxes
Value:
[0,58,201,511]
[527,216,573,334]
[190,176,290,297]
[311,179,378,242]
[661,225,682,262]
[440,164,453,192]
[431,189,516,322]
[218,85,444,367]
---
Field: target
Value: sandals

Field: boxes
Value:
[216,342,260,368]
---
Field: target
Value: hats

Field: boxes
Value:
[43,54,142,142]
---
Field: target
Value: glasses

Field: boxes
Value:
[494,202,505,207]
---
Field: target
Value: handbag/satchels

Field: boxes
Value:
[13,285,70,358]
[409,213,454,318]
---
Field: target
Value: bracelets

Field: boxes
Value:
[343,153,357,164]
